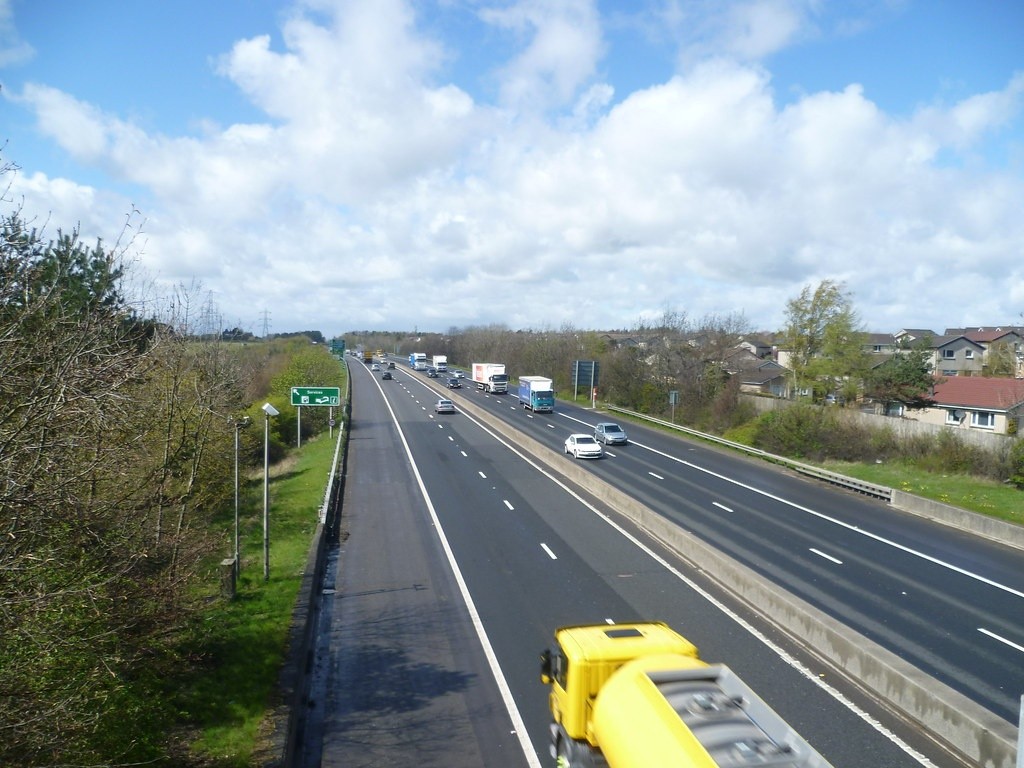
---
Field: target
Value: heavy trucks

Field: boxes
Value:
[409,352,426,371]
[472,362,510,394]
[538,618,835,768]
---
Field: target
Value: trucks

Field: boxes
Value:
[433,355,448,372]
[518,376,558,414]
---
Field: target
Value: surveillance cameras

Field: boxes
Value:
[261,402,279,415]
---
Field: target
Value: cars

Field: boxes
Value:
[446,378,462,389]
[564,433,603,458]
[453,370,465,378]
[425,365,438,378]
[380,358,388,364]
[345,348,388,363]
[434,399,455,413]
[372,364,380,371]
[388,362,396,369]
[381,372,392,379]
[594,423,628,446]
